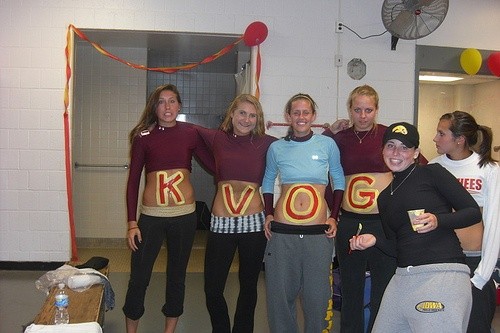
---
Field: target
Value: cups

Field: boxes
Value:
[67,275,101,288]
[407,209,425,231]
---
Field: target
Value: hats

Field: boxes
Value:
[382,122,419,150]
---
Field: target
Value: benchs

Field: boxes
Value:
[32,260,110,333]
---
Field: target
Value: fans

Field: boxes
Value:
[381,0,449,50]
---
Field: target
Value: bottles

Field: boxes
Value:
[54,283,69,325]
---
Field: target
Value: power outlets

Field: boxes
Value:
[335,22,344,33]
[335,55,343,66]
